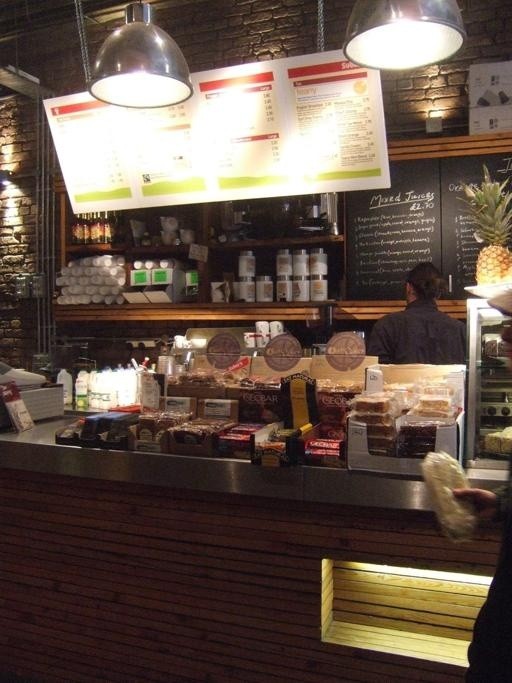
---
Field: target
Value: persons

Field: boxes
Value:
[429,437,510,680]
[364,260,469,369]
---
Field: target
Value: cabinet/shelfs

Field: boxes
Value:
[41,131,512,469]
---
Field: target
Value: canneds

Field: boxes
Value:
[232,246,329,302]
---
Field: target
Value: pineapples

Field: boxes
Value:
[455,164,512,286]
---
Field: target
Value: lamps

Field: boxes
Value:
[88,0,193,109]
[344,0,466,72]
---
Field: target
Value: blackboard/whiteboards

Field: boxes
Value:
[344,152,512,302]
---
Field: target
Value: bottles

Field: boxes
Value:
[56,356,156,412]
[209,247,329,303]
[141,230,151,246]
[69,211,123,243]
[54,254,191,303]
[328,222,339,235]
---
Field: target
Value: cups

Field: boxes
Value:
[157,356,185,384]
[243,321,284,348]
[159,216,196,245]
